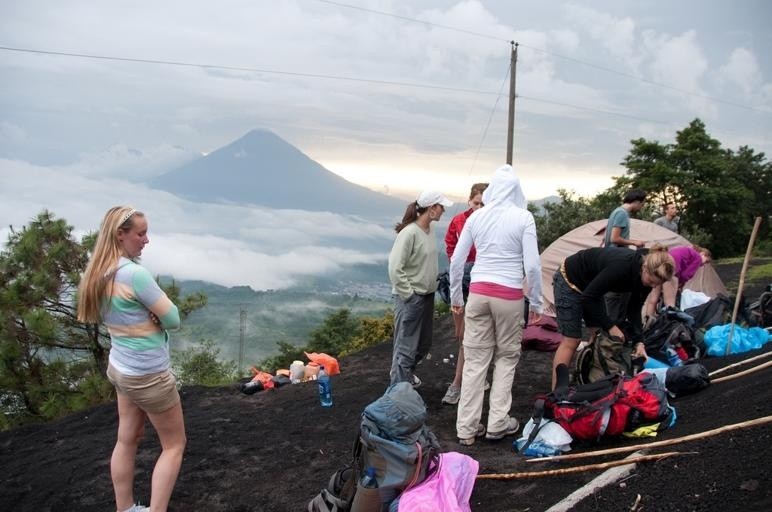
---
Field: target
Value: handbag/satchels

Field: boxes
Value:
[574,331,633,387]
[643,306,696,369]
[685,292,772,335]
[518,371,675,455]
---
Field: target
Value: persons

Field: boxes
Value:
[448,163,546,446]
[604,190,648,249]
[77,206,188,512]
[653,201,678,234]
[387,189,455,388]
[646,245,712,317]
[440,182,490,405]
[550,243,676,392]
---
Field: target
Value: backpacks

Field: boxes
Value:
[307,381,480,512]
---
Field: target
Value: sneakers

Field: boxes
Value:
[441,382,461,405]
[458,423,487,446]
[115,503,151,512]
[483,378,490,391]
[485,416,520,440]
[410,375,422,388]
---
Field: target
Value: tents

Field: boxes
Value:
[522,218,727,319]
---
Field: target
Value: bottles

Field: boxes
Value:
[360,465,380,491]
[289,360,320,380]
[317,365,333,408]
[666,349,684,367]
[511,438,561,458]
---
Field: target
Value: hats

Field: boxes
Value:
[416,189,454,208]
